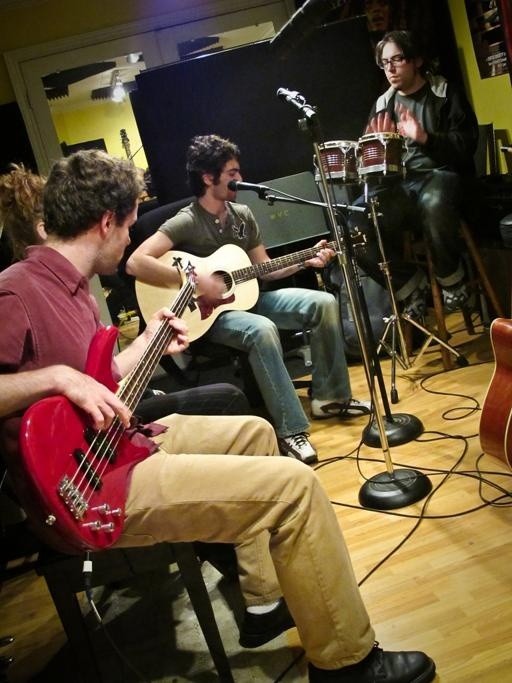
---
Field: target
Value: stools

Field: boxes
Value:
[35,546,235,683]
[401,217,505,369]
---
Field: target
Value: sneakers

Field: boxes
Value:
[310,396,374,420]
[280,432,317,464]
[402,276,434,321]
[442,259,471,312]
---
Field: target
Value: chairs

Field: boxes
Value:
[136,196,311,410]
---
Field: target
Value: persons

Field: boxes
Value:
[124,132,375,463]
[0,150,436,683]
[338,29,481,317]
[0,161,255,425]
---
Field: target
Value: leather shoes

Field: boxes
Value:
[239,597,295,648]
[309,639,436,683]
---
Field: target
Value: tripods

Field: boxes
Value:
[363,181,468,404]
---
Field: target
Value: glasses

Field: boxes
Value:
[377,56,408,71]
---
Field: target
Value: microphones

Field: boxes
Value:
[228,180,269,191]
[276,87,318,121]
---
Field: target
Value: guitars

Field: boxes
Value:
[479,145,512,469]
[120,128,150,206]
[135,227,367,342]
[1,258,196,552]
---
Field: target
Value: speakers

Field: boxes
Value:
[235,170,332,260]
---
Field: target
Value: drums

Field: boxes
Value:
[357,132,403,178]
[314,140,358,185]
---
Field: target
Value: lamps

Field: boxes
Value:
[111,70,126,103]
